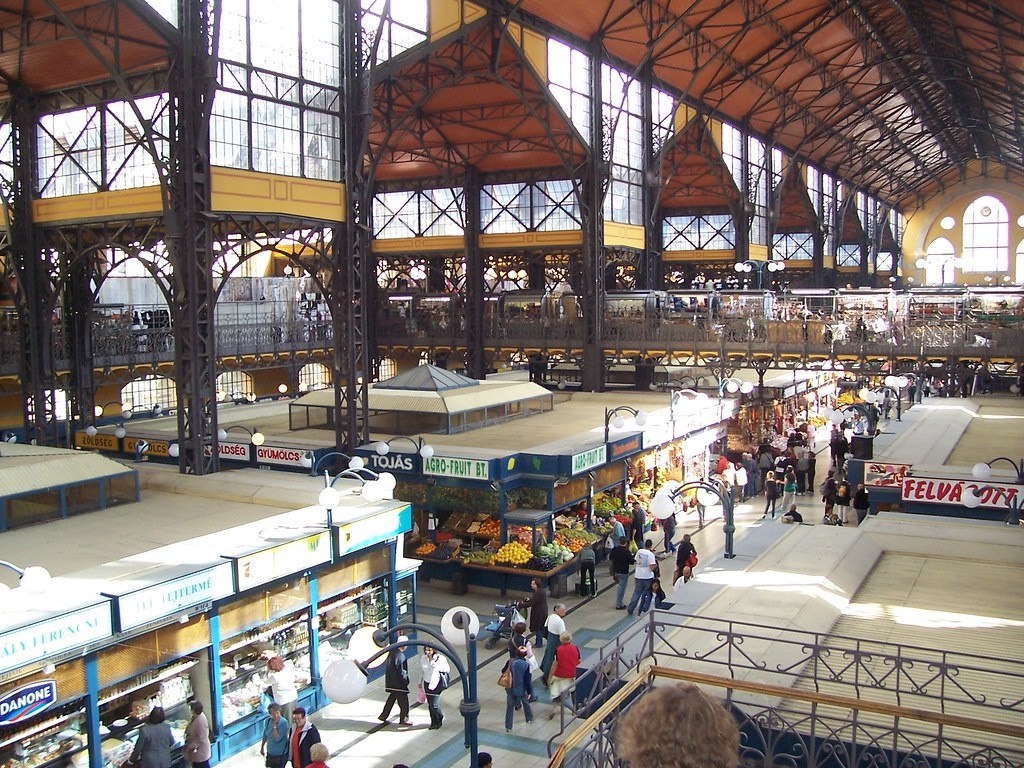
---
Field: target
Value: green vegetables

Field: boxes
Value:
[556,527,599,542]
[595,497,634,518]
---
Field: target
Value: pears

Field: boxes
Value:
[489,541,533,565]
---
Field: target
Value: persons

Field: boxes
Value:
[377,635,413,727]
[723,483,731,522]
[128,706,174,768]
[573,501,587,518]
[696,500,705,527]
[546,632,582,719]
[818,415,869,526]
[418,639,450,730]
[609,535,662,617]
[183,700,212,768]
[539,603,567,686]
[517,578,549,647]
[502,646,532,732]
[712,420,817,523]
[478,752,493,768]
[907,384,917,404]
[507,623,538,710]
[620,683,740,768]
[263,656,299,729]
[576,542,597,597]
[883,395,891,420]
[608,501,678,560]
[673,534,697,586]
[674,566,693,590]
[259,702,329,768]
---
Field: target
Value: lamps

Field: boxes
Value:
[217,427,265,462]
[562,373,584,385]
[960,485,1024,525]
[0,559,52,616]
[86,422,127,451]
[823,376,909,437]
[1010,383,1024,397]
[542,378,565,390]
[677,376,712,389]
[719,376,754,406]
[670,388,708,422]
[604,405,646,443]
[123,403,163,419]
[888,276,914,287]
[278,380,332,397]
[300,450,365,477]
[95,402,132,417]
[651,477,735,559]
[915,257,964,285]
[648,375,687,392]
[375,435,435,477]
[972,456,1024,485]
[358,374,378,384]
[734,258,786,289]
[216,391,257,404]
[317,467,397,526]
[984,274,1011,287]
[320,605,483,768]
[134,438,179,464]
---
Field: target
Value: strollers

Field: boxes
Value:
[484,598,529,649]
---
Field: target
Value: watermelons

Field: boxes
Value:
[538,539,575,562]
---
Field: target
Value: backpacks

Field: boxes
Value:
[838,485,847,498]
[820,480,831,498]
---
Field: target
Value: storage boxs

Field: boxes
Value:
[326,603,359,629]
[574,579,598,597]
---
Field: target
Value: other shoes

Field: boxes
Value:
[629,611,633,614]
[436,715,443,729]
[399,721,413,726]
[378,716,386,722]
[506,696,556,732]
[616,605,626,610]
[429,725,435,730]
[532,644,543,648]
[592,593,597,597]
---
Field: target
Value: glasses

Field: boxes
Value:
[294,717,302,722]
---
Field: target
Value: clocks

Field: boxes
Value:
[981,207,991,217]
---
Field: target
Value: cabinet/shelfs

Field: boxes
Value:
[317,588,389,677]
[0,708,89,768]
[219,611,311,727]
[96,661,200,768]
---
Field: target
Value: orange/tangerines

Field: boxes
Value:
[415,543,436,555]
[555,537,585,554]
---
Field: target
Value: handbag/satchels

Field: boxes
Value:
[542,614,551,639]
[257,693,273,713]
[689,554,698,567]
[417,682,427,704]
[672,571,679,585]
[498,658,512,688]
[605,535,614,549]
[510,609,525,629]
[628,540,638,553]
[519,608,527,620]
[524,655,539,673]
